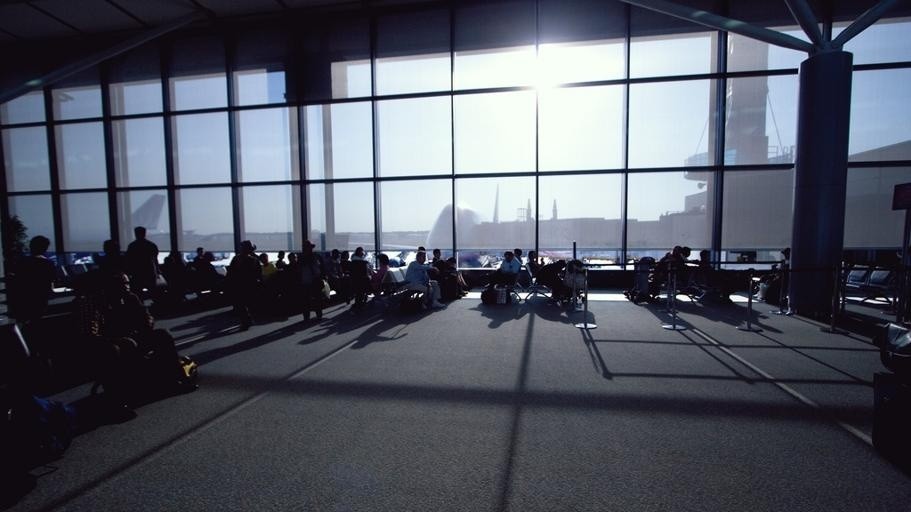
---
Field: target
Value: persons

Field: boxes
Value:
[653,245,790,305]
[481,247,569,304]
[24,227,469,324]
[77,270,200,418]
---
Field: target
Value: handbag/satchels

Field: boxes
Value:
[758,282,772,299]
[319,278,330,300]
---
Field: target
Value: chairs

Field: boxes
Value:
[60,258,563,322]
[635,262,899,313]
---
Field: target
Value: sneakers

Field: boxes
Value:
[432,300,446,308]
